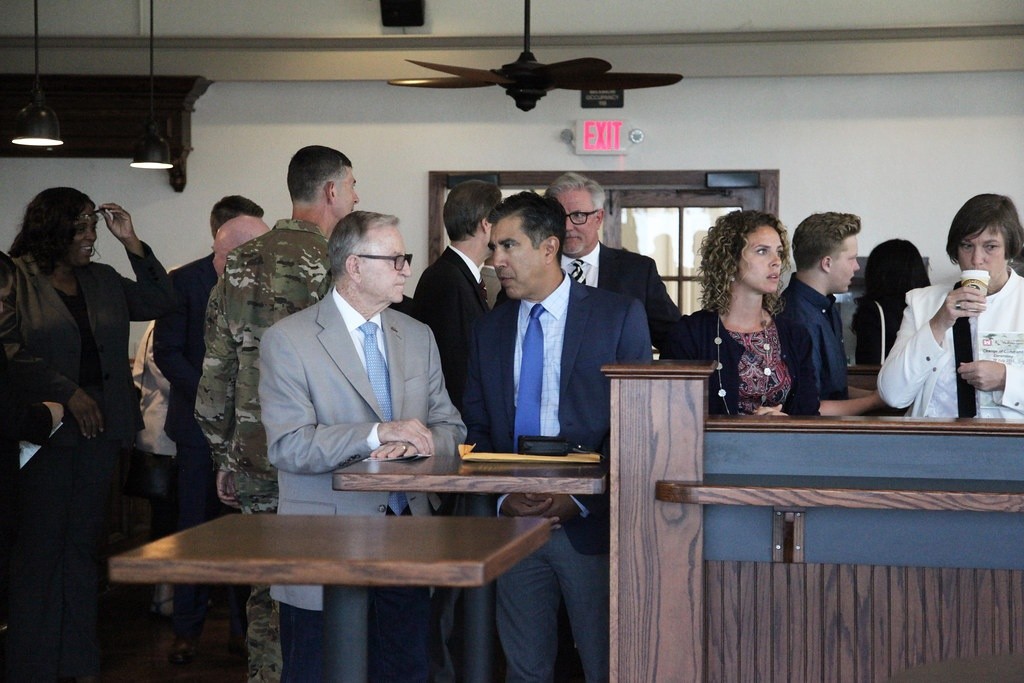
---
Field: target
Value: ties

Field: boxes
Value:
[479,278,488,306]
[570,260,586,286]
[513,304,545,453]
[359,322,409,516]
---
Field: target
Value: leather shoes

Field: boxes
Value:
[226,634,248,665]
[168,637,198,662]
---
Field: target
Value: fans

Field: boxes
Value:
[387,0,683,112]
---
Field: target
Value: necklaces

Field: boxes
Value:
[714,309,774,416]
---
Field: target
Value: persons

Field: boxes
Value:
[155,195,272,668]
[393,169,696,683]
[196,145,359,683]
[878,193,1024,423]
[685,210,818,417]
[0,186,185,682]
[853,238,928,367]
[795,212,883,416]
[250,211,466,683]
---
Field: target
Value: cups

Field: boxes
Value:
[960,270,990,311]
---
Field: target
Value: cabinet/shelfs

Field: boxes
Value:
[0,74,215,192]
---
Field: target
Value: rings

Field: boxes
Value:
[953,301,963,311]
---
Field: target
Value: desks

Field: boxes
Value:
[108,513,549,683]
[332,455,607,683]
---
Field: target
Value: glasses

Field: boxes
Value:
[565,209,599,224]
[345,254,413,271]
[76,208,105,224]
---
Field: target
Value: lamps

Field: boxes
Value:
[131,0,173,169]
[9,0,64,147]
[562,117,645,157]
